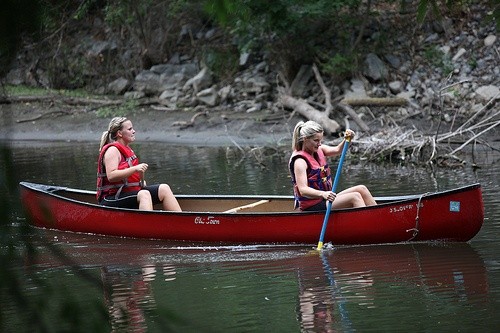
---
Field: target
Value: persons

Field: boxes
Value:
[288,120,377,211]
[96,117,182,211]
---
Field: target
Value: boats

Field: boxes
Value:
[18,181,486,245]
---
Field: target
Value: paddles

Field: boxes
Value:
[313,131,355,254]
[317,254,349,328]
[140,168,148,190]
